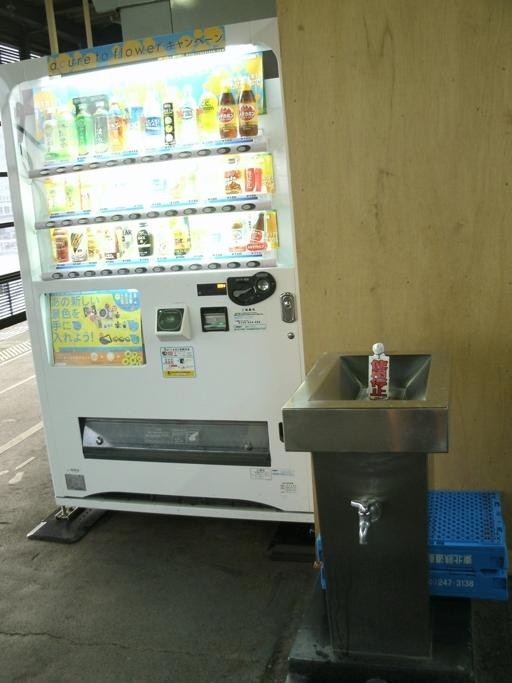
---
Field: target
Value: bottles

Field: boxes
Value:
[217,80,237,139]
[40,151,279,265]
[236,81,259,138]
[38,78,218,165]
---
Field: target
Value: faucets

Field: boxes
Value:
[351,494,382,546]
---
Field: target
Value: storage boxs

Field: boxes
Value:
[315,490,508,602]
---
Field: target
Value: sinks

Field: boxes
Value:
[281,350,454,454]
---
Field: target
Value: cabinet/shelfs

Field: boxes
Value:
[1,17,321,561]
